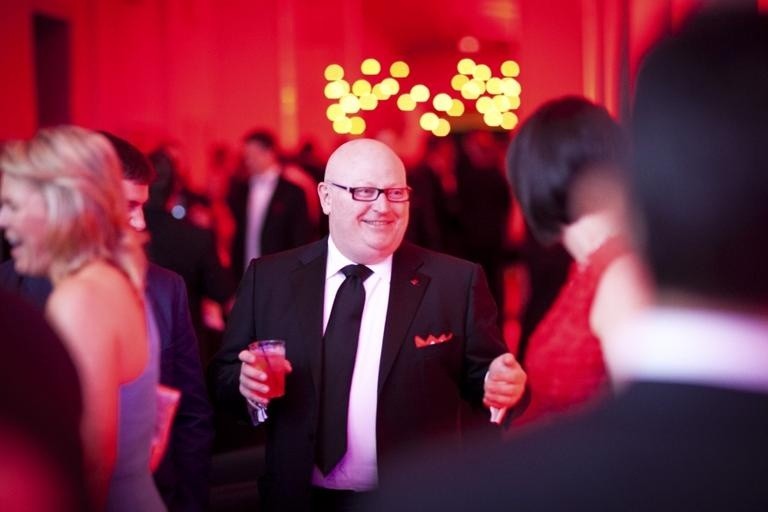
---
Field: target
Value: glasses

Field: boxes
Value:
[325,179,412,203]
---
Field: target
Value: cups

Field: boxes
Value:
[248,338,286,398]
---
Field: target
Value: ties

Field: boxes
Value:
[315,265,373,477]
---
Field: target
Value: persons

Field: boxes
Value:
[0,125,529,511]
[364,1,767,512]
